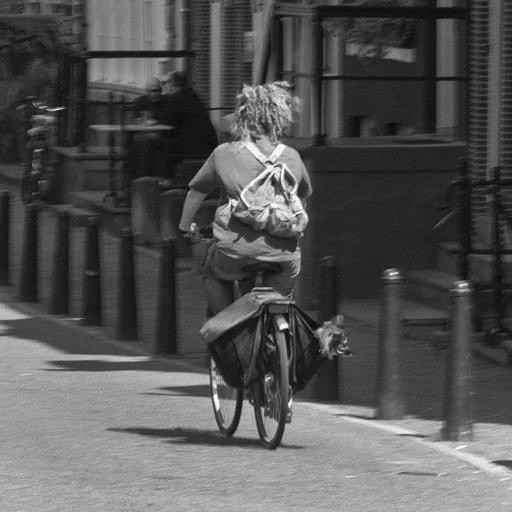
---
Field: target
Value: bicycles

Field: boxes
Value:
[178,217,296,448]
[16,95,67,203]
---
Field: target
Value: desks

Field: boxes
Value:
[87,124,177,208]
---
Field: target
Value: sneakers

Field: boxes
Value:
[262,376,293,424]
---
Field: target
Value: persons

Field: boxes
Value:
[179,82,313,316]
[131,77,169,125]
[142,71,218,176]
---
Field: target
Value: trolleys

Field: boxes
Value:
[100,92,147,210]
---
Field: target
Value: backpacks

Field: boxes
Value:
[197,284,340,397]
[230,139,310,238]
